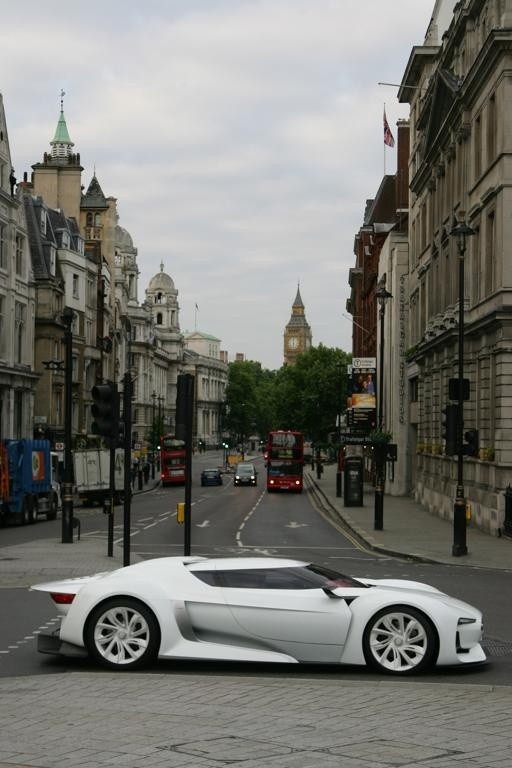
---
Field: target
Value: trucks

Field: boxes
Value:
[72,446,136,505]
[0,437,62,526]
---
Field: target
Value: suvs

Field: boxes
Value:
[234,462,259,486]
[200,468,223,486]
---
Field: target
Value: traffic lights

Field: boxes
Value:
[441,403,459,455]
[464,427,480,459]
[113,418,124,449]
[88,381,117,438]
[176,502,185,523]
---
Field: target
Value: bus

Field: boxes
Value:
[159,431,187,486]
[266,429,305,494]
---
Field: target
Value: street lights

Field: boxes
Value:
[54,307,80,543]
[335,360,345,497]
[451,219,475,557]
[371,276,393,531]
[222,441,226,466]
[225,444,228,468]
[151,390,165,479]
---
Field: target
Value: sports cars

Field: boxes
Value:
[27,553,492,673]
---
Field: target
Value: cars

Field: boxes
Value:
[264,451,268,458]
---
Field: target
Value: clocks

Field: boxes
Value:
[286,335,301,351]
